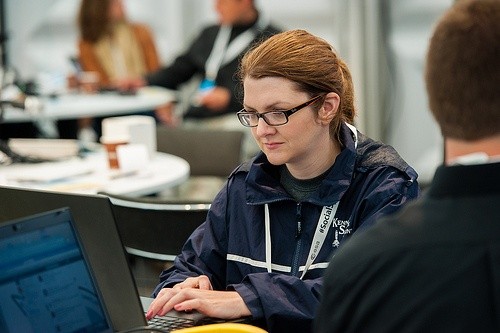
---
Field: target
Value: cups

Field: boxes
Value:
[80,81,97,96]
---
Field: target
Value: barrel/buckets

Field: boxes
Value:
[100,116,157,175]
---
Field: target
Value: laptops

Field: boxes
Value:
[0,185,226,333]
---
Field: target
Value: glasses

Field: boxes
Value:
[237,93,327,127]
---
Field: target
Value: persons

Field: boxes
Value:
[313,0,500,333]
[77,0,283,146]
[143,29,418,333]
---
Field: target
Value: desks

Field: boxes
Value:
[2,88,176,132]
[0,158,224,296]
